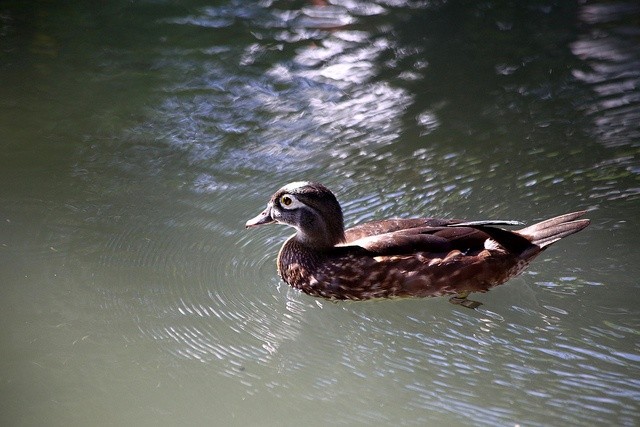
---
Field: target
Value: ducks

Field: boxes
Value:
[245,181,590,308]
